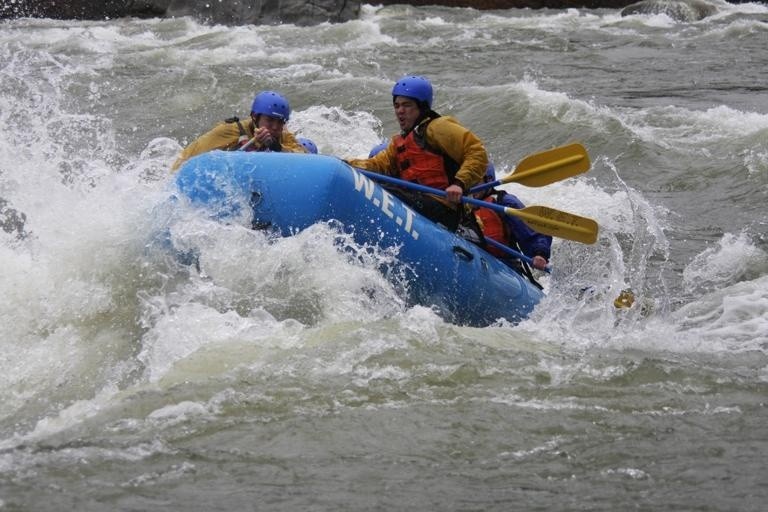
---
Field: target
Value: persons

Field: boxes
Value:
[344,74,489,231]
[473,163,552,270]
[172,90,303,173]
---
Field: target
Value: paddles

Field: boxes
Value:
[463,144,590,196]
[457,223,655,318]
[351,165,598,245]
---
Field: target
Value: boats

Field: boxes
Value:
[148,147,549,330]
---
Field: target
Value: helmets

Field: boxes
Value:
[296,138,318,155]
[391,75,433,110]
[485,161,495,183]
[250,90,290,122]
[368,143,387,158]
